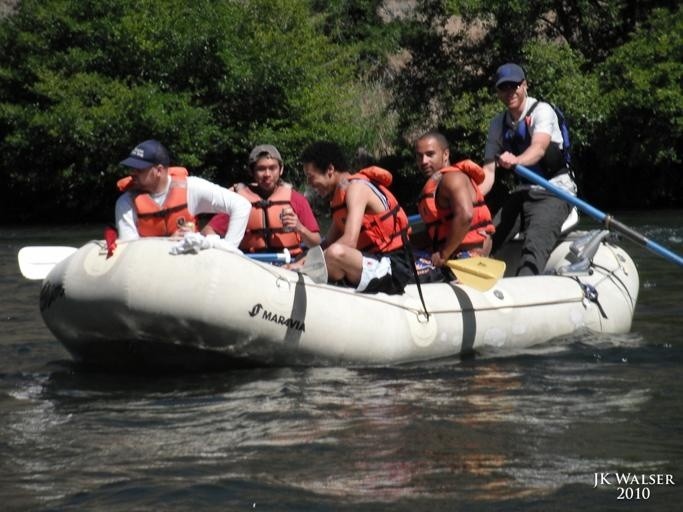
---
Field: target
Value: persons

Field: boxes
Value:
[281,141,415,295]
[201,144,322,268]
[477,65,577,276]
[405,130,496,284]
[112,138,251,256]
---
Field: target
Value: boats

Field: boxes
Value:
[37,224,642,368]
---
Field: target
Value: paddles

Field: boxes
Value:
[16,244,291,281]
[296,245,329,280]
[409,244,507,293]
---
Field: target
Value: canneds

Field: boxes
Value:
[184,220,195,233]
[281,208,293,233]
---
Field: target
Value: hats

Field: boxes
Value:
[119,139,170,171]
[246,143,284,165]
[492,63,526,90]
